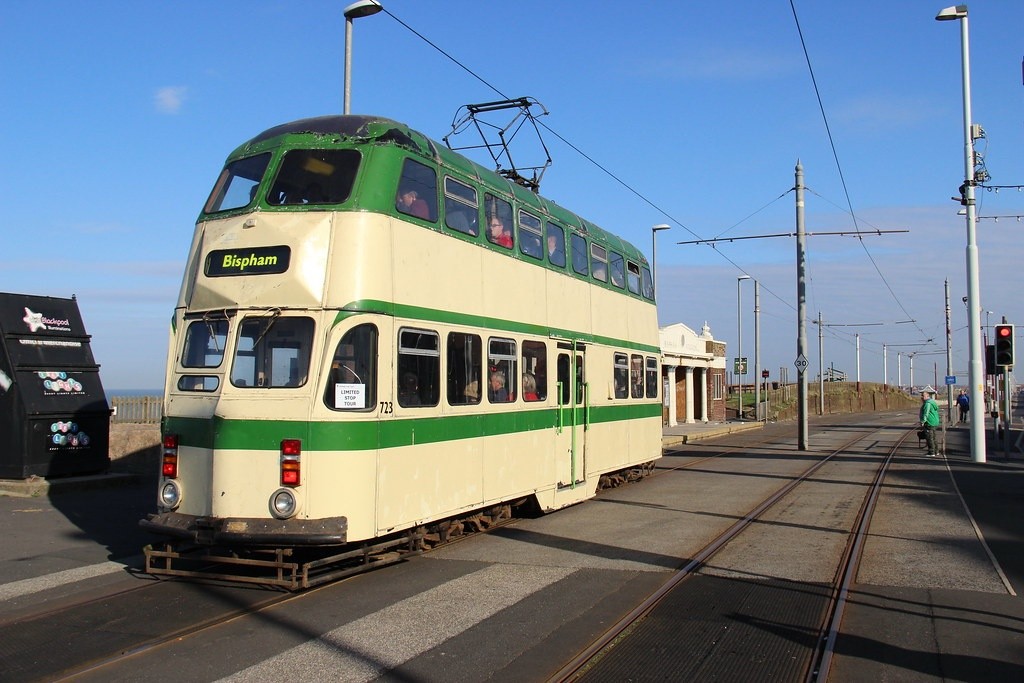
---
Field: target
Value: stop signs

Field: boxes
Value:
[762,371,769,378]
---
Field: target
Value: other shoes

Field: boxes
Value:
[935,452,939,455]
[925,453,935,457]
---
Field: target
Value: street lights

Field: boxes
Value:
[343,0,384,115]
[937,5,987,463]
[737,275,751,418]
[651,224,671,300]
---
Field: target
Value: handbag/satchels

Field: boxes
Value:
[917,430,926,440]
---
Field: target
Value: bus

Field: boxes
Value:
[136,115,664,591]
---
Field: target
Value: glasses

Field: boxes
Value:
[486,222,501,228]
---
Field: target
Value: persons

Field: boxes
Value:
[488,216,513,249]
[489,372,509,402]
[984,391,992,414]
[919,392,940,457]
[398,372,421,405]
[536,235,565,266]
[397,184,430,220]
[614,367,657,398]
[446,200,469,232]
[522,373,539,402]
[954,390,969,423]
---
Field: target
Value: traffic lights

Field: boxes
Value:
[995,324,1015,366]
